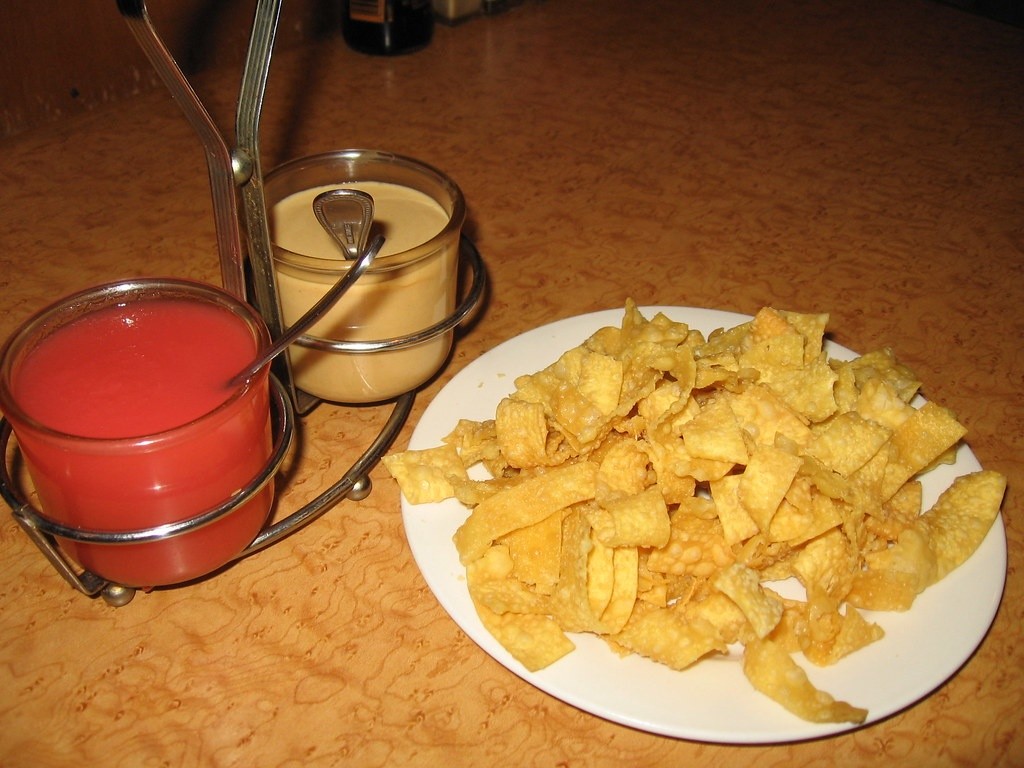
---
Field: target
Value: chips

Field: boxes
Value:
[378,296,1007,728]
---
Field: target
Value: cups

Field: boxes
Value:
[260,147,467,404]
[0,277,275,587]
[434,0,480,27]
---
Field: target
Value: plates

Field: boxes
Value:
[400,303,1006,743]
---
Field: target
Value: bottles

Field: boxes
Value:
[339,0,436,57]
